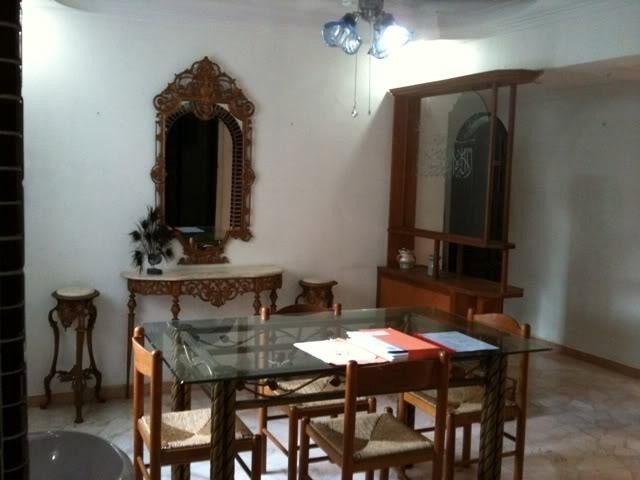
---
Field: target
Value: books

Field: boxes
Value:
[292,335,390,371]
[342,326,441,365]
[409,329,501,356]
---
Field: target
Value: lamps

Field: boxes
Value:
[322,0,412,58]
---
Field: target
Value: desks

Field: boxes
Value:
[121,260,287,411]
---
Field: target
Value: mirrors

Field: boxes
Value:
[149,55,256,263]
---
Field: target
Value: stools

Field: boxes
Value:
[39,287,105,422]
[296,275,338,307]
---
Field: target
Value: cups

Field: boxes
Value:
[428,254,441,277]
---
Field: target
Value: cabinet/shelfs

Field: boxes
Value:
[378,70,543,312]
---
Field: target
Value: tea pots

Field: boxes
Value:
[397,247,418,270]
